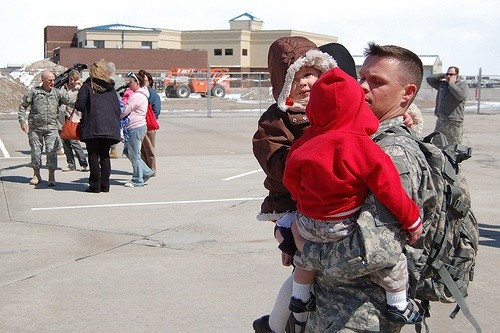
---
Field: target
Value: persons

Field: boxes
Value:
[18,60,161,193]
[426,66,468,150]
[316,43,358,82]
[295,40,424,332]
[283,67,423,324]
[252,35,413,333]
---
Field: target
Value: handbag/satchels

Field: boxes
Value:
[135,91,160,131]
[59,106,80,140]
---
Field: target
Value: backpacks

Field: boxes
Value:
[374,124,479,303]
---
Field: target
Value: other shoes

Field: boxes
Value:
[125,182,144,187]
[253,314,275,333]
[62,166,76,172]
[387,300,423,324]
[84,188,100,193]
[289,291,317,313]
[80,165,86,172]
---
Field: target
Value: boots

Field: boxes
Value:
[48,170,56,185]
[30,169,41,185]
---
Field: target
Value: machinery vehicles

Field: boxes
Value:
[163,67,230,98]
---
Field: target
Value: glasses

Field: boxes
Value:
[446,72,457,76]
